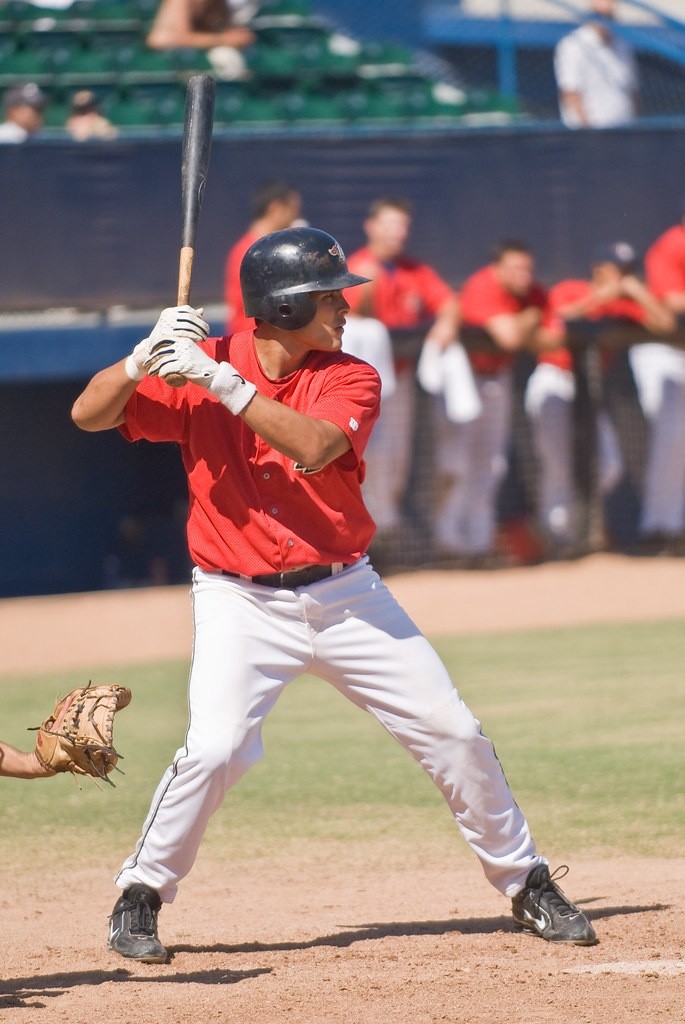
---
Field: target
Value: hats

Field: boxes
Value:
[600,243,638,266]
[8,83,46,108]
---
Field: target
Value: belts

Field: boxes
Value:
[222,563,348,591]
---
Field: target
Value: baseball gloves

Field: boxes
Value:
[24,679,133,793]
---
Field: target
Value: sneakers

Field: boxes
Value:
[108,882,167,964]
[511,863,597,946]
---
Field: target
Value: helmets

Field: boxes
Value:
[239,227,372,330]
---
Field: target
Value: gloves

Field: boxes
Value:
[125,305,210,382]
[143,337,257,416]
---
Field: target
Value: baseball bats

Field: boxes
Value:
[164,70,217,389]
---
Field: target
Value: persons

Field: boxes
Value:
[0,685,132,779]
[525,255,677,552]
[0,86,47,145]
[432,240,564,561]
[340,204,460,563]
[223,187,302,335]
[145,0,253,48]
[69,226,596,964]
[67,91,113,138]
[554,0,644,128]
[626,221,685,539]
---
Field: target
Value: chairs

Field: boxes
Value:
[0,0,519,134]
